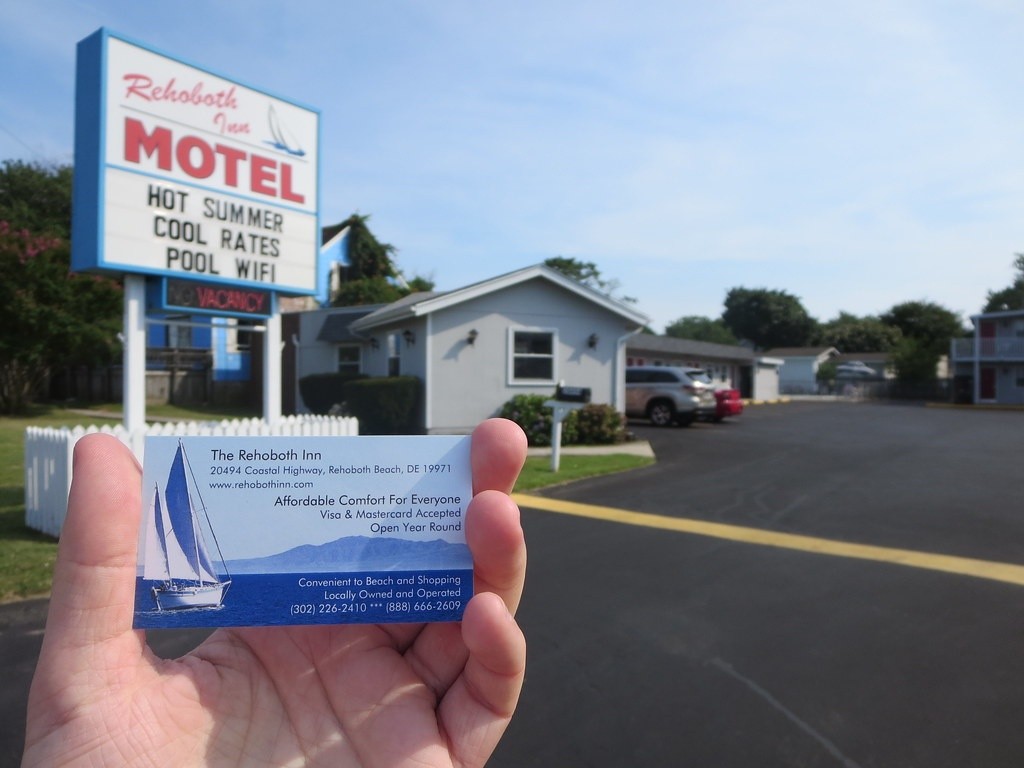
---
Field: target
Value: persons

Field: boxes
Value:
[22,414,528,768]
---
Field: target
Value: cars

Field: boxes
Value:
[713,377,744,424]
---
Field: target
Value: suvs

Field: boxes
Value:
[625,364,719,428]
